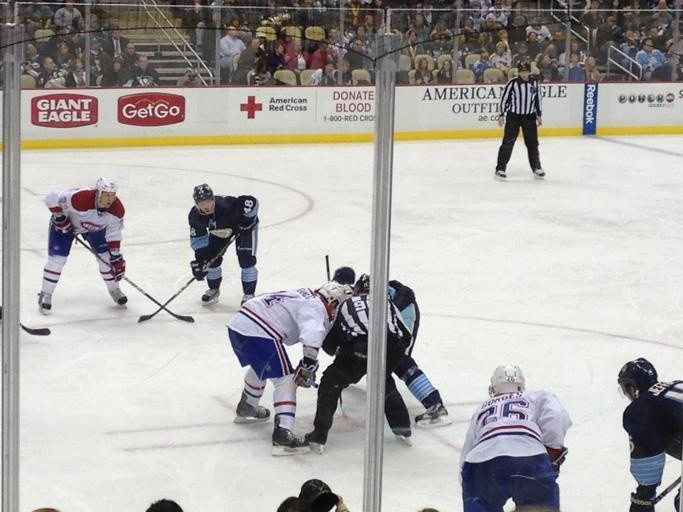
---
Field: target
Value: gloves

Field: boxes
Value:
[629,502,655,512]
[293,356,320,387]
[54,215,73,235]
[111,255,127,283]
[236,225,249,238]
[191,259,210,281]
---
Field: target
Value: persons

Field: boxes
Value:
[331,267,355,285]
[37,177,127,315]
[225,281,347,456]
[354,274,451,428]
[145,499,184,511]
[188,184,259,308]
[306,288,413,454]
[618,359,683,512]
[495,63,546,181]
[459,363,572,512]
[277,494,349,512]
[1,0,159,89]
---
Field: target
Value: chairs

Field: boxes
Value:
[274,70,297,85]
[465,54,482,68]
[483,68,505,84]
[408,70,420,84]
[300,69,318,87]
[399,55,411,72]
[305,25,326,42]
[414,54,434,71]
[437,54,452,69]
[34,29,56,42]
[232,53,241,71]
[508,68,518,82]
[246,70,272,85]
[281,27,302,47]
[432,68,441,85]
[20,74,37,88]
[256,26,277,42]
[352,69,372,86]
[456,68,475,84]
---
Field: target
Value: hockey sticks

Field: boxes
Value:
[74,236,194,324]
[138,236,238,323]
[325,255,351,420]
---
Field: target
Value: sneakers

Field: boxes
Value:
[272,428,310,446]
[534,168,546,178]
[38,291,53,309]
[495,169,507,178]
[108,288,128,305]
[235,400,270,418]
[414,402,449,422]
[305,430,322,442]
[241,294,255,307]
[201,288,219,301]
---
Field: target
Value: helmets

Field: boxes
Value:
[190,184,217,203]
[518,60,530,71]
[617,357,659,402]
[489,362,525,395]
[96,177,120,196]
[316,280,347,305]
